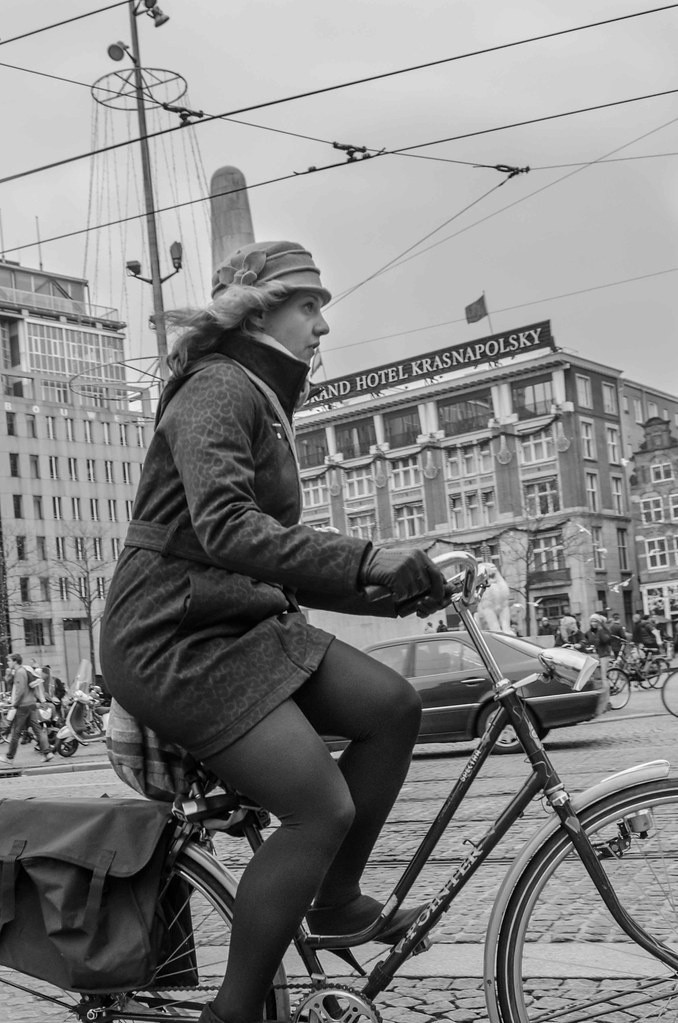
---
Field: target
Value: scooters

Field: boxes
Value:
[54,690,111,758]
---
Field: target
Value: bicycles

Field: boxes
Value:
[0,550,677,1023]
[604,635,671,695]
[660,669,678,718]
[0,708,16,744]
[586,645,632,711]
[614,634,660,696]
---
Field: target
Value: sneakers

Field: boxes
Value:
[40,752,54,762]
[0,757,13,765]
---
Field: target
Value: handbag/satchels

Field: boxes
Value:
[0,798,201,994]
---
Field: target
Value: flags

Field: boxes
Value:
[465,294,487,324]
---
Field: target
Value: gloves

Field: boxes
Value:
[397,583,457,619]
[355,545,447,605]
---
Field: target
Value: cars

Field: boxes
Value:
[317,630,611,754]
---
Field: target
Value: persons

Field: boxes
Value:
[0,652,55,765]
[96,237,457,1023]
[35,676,112,753]
[424,612,678,694]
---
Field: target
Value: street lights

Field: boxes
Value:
[105,0,184,398]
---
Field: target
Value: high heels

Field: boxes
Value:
[197,1002,223,1023]
[304,895,444,976]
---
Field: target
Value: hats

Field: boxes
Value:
[210,240,331,307]
[591,613,602,624]
